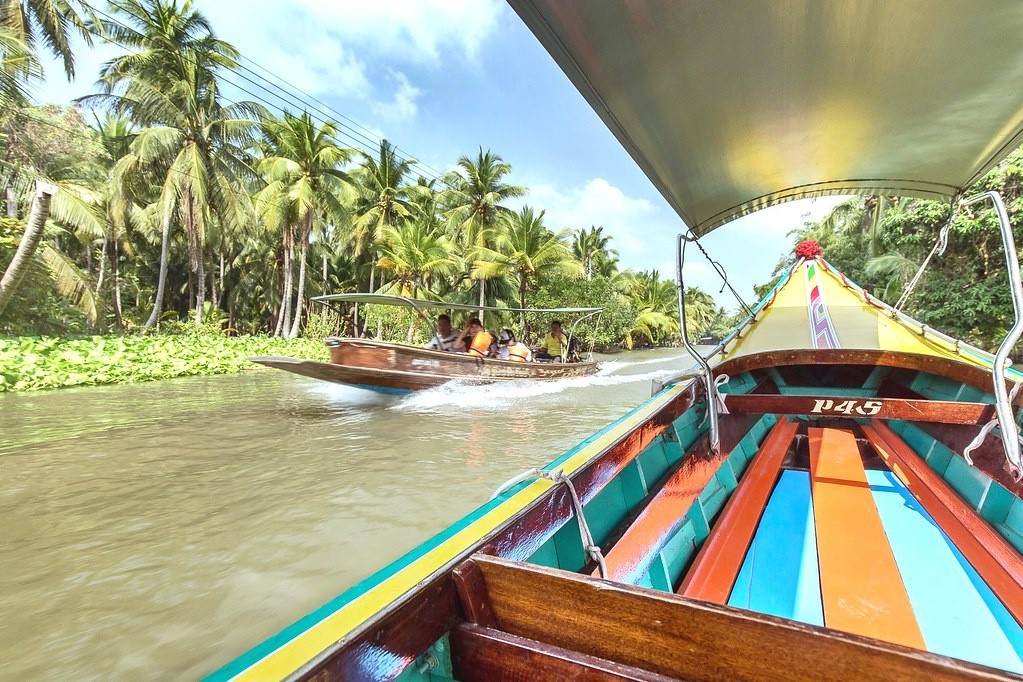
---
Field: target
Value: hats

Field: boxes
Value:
[488,330,498,341]
[468,318,481,326]
[499,329,514,344]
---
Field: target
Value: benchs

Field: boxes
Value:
[449,551,1023,682]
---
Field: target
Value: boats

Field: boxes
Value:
[249,293,605,396]
[202,0,1023,682]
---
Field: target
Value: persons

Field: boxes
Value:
[535,321,567,363]
[426,314,464,352]
[453,318,492,357]
[486,329,532,363]
